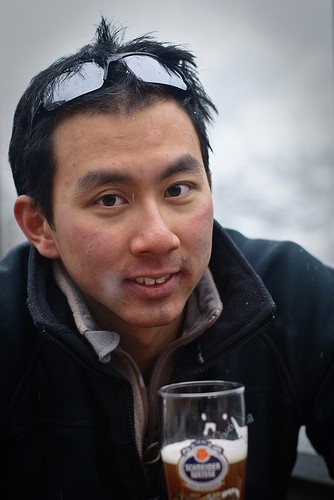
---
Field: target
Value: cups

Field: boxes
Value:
[158,381,249,500]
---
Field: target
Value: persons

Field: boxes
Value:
[1,15,333,500]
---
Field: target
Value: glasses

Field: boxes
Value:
[30,50,196,130]
